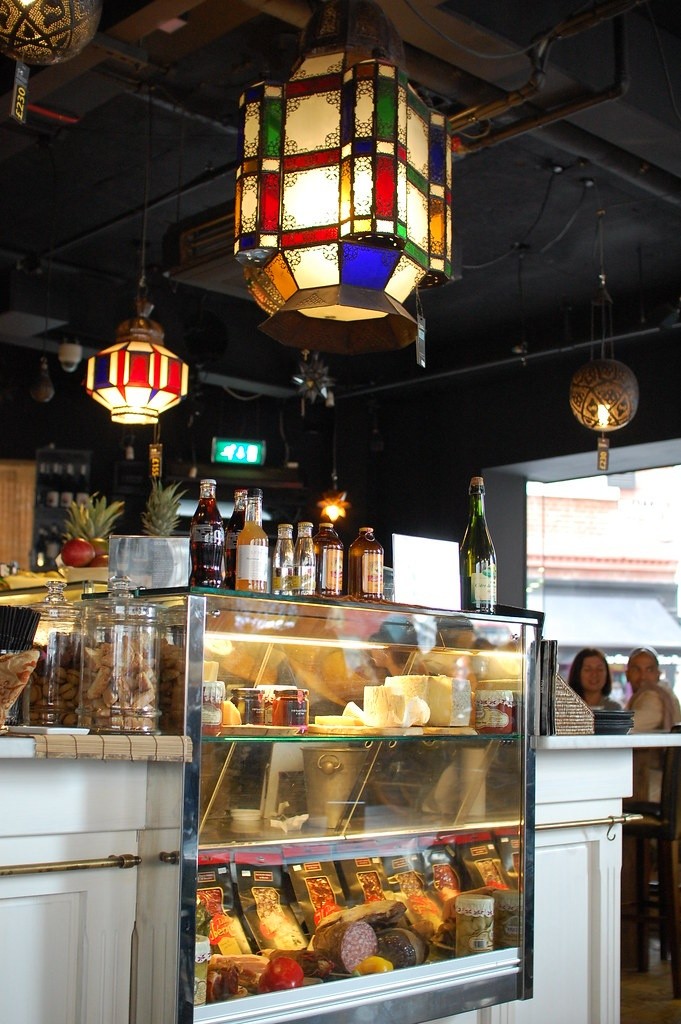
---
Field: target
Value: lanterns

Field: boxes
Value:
[570,359,640,430]
[236,0,455,356]
[84,317,189,424]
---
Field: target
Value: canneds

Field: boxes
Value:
[199,682,309,738]
[474,690,513,734]
[454,890,522,957]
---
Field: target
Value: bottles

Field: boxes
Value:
[36,522,61,571]
[225,490,268,594]
[273,524,294,597]
[35,462,90,507]
[160,598,188,735]
[459,478,496,615]
[294,522,316,597]
[348,527,384,601]
[77,575,162,736]
[189,479,224,589]
[24,580,106,728]
[313,523,344,597]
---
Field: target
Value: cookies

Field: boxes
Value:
[26,638,156,732]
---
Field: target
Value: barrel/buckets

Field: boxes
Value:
[301,746,369,828]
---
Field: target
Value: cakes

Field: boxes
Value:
[362,674,472,726]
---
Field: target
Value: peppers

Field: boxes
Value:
[352,956,393,976]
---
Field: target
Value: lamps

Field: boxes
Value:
[233,0,452,354]
[569,208,638,431]
[0,0,104,66]
[31,261,54,402]
[87,142,190,427]
[294,351,334,405]
[318,404,351,522]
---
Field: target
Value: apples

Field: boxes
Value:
[61,537,109,567]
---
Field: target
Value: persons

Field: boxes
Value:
[625,646,680,734]
[568,648,623,712]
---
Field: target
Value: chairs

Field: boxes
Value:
[622,726,681,1000]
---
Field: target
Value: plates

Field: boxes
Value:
[59,567,108,581]
[592,711,635,735]
[230,810,261,821]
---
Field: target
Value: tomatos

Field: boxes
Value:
[260,956,304,990]
